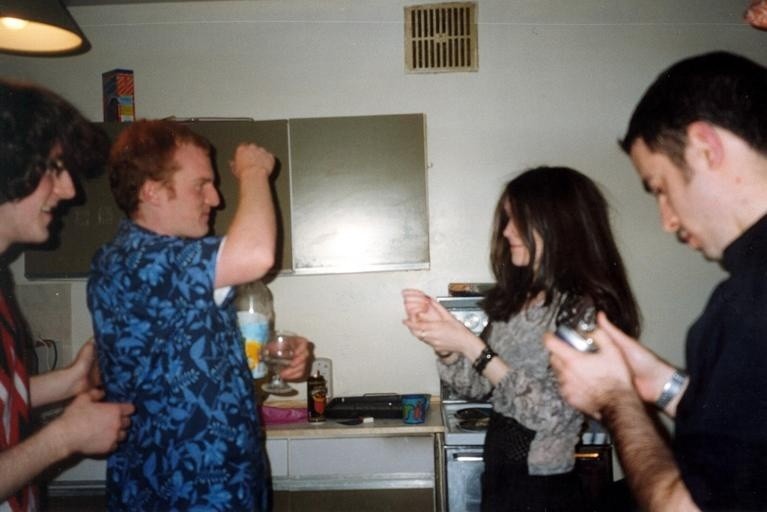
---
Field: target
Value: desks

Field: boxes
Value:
[43,395,449,511]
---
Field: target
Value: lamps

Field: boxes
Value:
[0,0,93,59]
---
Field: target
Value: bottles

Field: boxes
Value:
[307,370,327,424]
[232,278,272,381]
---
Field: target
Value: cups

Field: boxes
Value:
[401,395,430,425]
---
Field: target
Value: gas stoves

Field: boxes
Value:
[437,398,617,445]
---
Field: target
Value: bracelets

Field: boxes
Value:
[652,366,686,412]
[470,347,496,373]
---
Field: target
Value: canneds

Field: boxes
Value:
[307,375,328,425]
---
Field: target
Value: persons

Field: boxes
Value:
[400,164,641,511]
[0,80,136,512]
[540,51,767,512]
[83,117,316,512]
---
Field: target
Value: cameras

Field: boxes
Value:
[554,308,607,351]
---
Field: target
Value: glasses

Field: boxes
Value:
[41,158,65,177]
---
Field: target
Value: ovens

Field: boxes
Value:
[445,444,614,510]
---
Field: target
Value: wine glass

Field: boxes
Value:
[261,331,299,395]
